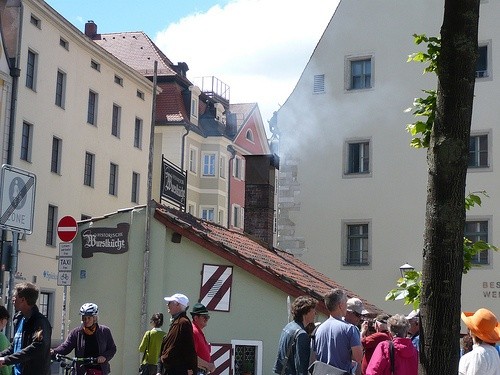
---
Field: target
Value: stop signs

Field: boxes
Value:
[57,215,78,243]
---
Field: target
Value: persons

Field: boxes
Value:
[51,303,117,375]
[139,293,217,375]
[459,308,500,375]
[274,288,427,375]
[0,281,52,375]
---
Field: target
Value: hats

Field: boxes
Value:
[376,313,389,324]
[346,298,369,314]
[164,293,189,307]
[405,309,420,321]
[461,308,500,343]
[190,302,210,315]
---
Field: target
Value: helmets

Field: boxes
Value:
[79,302,99,316]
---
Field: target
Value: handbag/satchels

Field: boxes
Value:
[139,363,149,375]
[309,361,350,375]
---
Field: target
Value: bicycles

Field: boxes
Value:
[52,352,98,375]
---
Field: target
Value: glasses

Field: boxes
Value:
[12,297,24,303]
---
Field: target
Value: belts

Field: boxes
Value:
[198,368,206,375]
